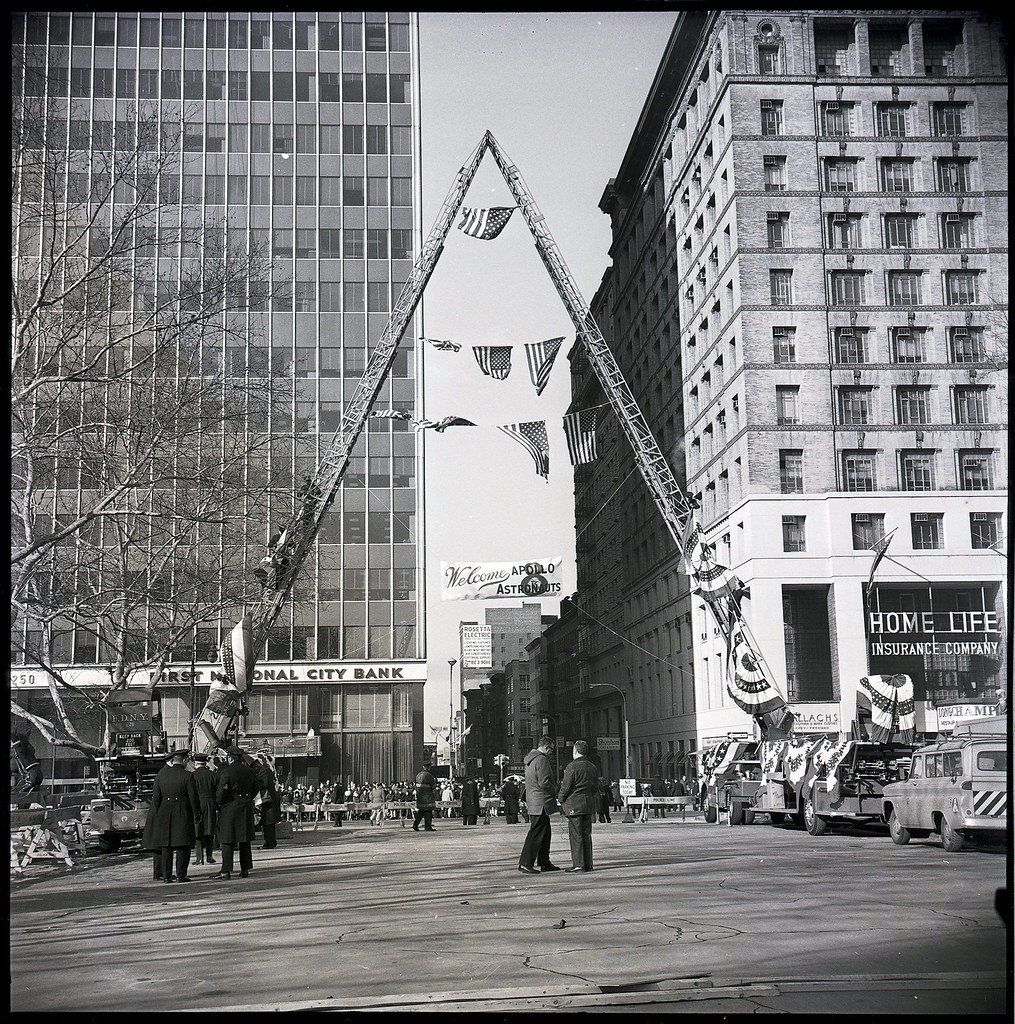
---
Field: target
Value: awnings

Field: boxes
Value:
[651,751,673,766]
[667,750,692,766]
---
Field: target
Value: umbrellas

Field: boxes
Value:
[503,774,525,782]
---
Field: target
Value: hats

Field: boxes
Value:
[172,749,190,757]
[165,753,175,760]
[195,754,208,762]
[227,746,244,758]
[249,759,260,768]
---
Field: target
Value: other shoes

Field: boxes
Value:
[412,826,419,831]
[257,844,274,850]
[425,827,436,831]
[152,876,164,881]
[192,860,204,866]
[370,819,373,826]
[207,857,216,863]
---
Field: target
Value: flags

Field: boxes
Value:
[457,205,515,241]
[371,409,599,485]
[418,336,564,398]
[220,613,253,691]
[866,535,893,608]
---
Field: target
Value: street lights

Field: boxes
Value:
[447,656,457,780]
[588,684,630,778]
[530,713,559,783]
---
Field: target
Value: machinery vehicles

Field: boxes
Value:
[488,139,923,836]
[89,132,486,855]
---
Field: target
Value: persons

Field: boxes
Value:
[10,733,43,810]
[557,739,601,873]
[502,776,530,824]
[381,777,419,820]
[343,780,386,826]
[462,775,505,826]
[277,779,343,827]
[141,746,281,883]
[435,778,463,819]
[598,774,700,823]
[412,762,437,830]
[517,736,560,874]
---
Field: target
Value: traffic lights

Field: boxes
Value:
[493,756,509,766]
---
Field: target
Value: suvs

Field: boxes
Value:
[879,734,1008,852]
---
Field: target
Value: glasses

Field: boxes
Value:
[548,746,554,751]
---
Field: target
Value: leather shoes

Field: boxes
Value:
[565,865,592,873]
[239,870,248,878]
[209,870,230,880]
[164,879,173,883]
[519,865,540,874]
[176,876,191,883]
[541,864,560,871]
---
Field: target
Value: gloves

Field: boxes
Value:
[194,812,203,825]
